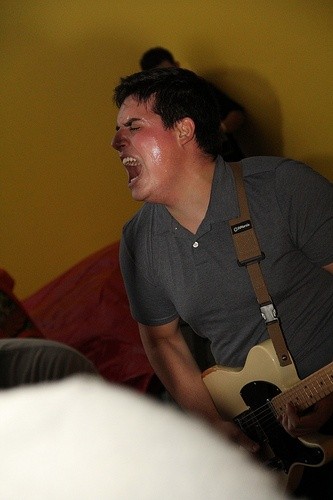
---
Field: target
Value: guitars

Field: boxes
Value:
[177,338,333,497]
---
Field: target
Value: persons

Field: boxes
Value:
[112,67,333,453]
[140,47,247,161]
